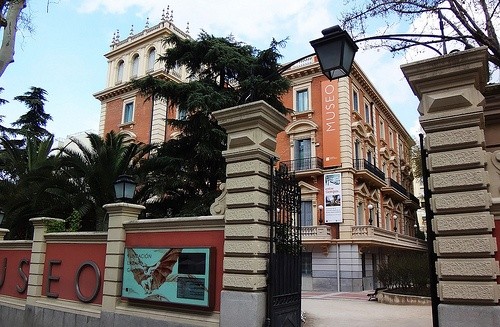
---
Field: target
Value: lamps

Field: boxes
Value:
[114,168,139,204]
[309,24,360,81]
[0,207,8,228]
